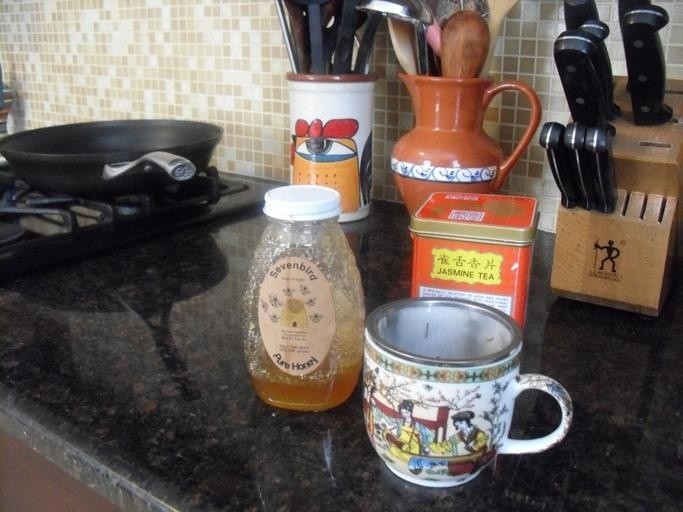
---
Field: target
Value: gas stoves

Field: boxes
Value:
[0,165,285,283]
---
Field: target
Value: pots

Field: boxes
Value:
[0,117,227,199]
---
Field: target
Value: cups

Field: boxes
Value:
[286,73,380,224]
[361,297,572,487]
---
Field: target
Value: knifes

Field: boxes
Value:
[584,122,618,213]
[580,20,616,116]
[553,30,607,123]
[538,121,581,208]
[617,0,674,127]
[566,120,599,210]
[562,0,600,31]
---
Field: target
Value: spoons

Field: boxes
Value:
[354,0,435,77]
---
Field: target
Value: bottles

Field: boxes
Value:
[244,184,365,413]
